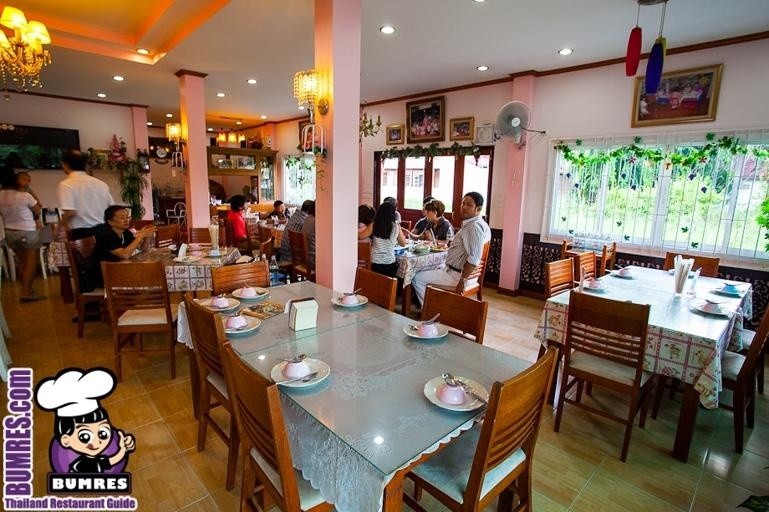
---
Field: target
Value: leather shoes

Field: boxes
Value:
[72,315,101,322]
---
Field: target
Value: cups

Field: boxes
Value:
[674,258,695,296]
[208,225,219,249]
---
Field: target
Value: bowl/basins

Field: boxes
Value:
[211,297,229,309]
[240,287,257,297]
[437,383,466,404]
[282,363,309,379]
[589,279,600,289]
[189,243,199,248]
[417,322,438,336]
[227,317,247,329]
[619,269,630,275]
[342,294,359,304]
[721,286,737,293]
[703,303,720,313]
[416,248,430,255]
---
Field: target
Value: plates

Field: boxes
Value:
[201,298,240,311]
[423,375,489,411]
[715,288,741,295]
[232,287,269,299]
[403,323,448,339]
[432,249,445,252]
[331,295,368,307]
[693,307,730,317]
[222,317,261,333]
[583,281,607,290]
[669,269,695,278]
[611,270,636,277]
[271,358,330,387]
[168,245,203,251]
[173,256,200,262]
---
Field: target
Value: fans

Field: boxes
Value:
[494,99,534,150]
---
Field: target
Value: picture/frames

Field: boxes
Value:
[404,95,447,143]
[236,157,255,171]
[628,61,725,129]
[449,116,474,141]
[88,148,113,168]
[386,124,406,145]
[474,122,500,145]
[217,158,235,170]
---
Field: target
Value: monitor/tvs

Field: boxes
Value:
[0,122,80,169]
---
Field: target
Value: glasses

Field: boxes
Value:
[114,216,132,222]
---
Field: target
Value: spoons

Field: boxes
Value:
[442,373,487,405]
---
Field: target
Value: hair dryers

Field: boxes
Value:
[284,298,318,332]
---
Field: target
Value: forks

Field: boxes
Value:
[276,372,318,386]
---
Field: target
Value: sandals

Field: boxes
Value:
[20,288,42,303]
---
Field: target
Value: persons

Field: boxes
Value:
[412,191,491,311]
[224,175,456,291]
[1,168,46,302]
[81,204,158,292]
[17,171,43,229]
[53,146,115,323]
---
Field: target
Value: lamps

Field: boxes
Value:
[0,5,56,91]
[290,65,328,115]
[626,1,668,96]
[217,128,245,143]
[160,121,188,146]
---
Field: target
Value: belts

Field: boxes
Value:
[447,262,461,274]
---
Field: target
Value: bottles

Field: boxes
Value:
[269,255,279,284]
[446,227,454,240]
[220,219,225,248]
